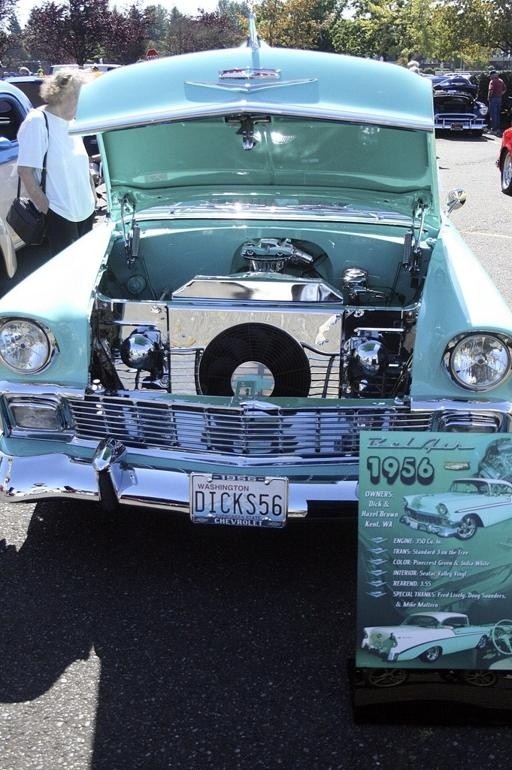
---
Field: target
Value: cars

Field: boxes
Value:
[431,73,489,137]
[1,74,55,165]
[400,478,512,541]
[1,10,511,523]
[497,127,512,195]
[361,612,491,665]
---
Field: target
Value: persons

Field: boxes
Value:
[14,70,96,259]
[0,55,115,81]
[396,57,512,137]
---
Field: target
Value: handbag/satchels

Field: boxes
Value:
[6,196,48,246]
